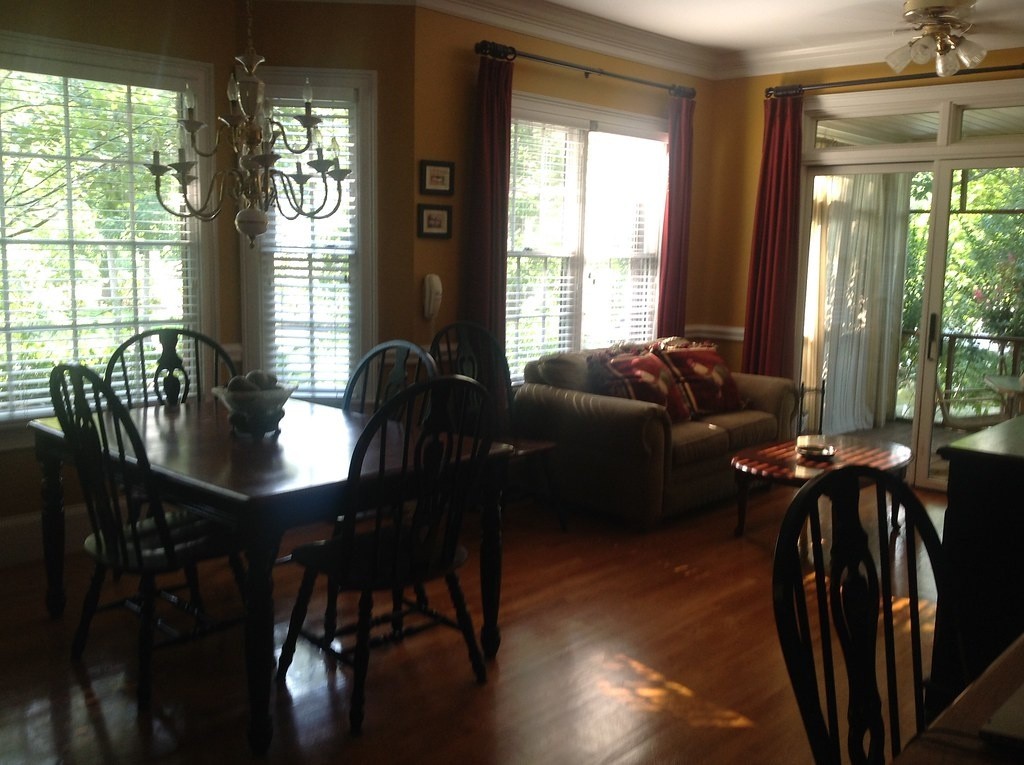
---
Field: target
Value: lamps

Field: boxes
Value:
[143,0,352,251]
[882,0,989,79]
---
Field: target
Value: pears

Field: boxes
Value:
[227,370,282,392]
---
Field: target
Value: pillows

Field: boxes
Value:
[585,342,691,408]
[606,347,692,426]
[661,339,739,416]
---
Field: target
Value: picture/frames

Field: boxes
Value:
[417,203,453,240]
[418,158,455,197]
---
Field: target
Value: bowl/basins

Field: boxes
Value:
[209,381,300,414]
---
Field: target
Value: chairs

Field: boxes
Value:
[770,463,962,765]
[936,380,1010,435]
[274,372,497,739]
[430,322,571,533]
[326,337,446,528]
[102,329,238,585]
[47,365,246,712]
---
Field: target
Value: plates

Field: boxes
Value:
[796,446,836,458]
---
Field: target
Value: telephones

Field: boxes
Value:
[424,273,442,322]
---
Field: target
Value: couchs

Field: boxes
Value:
[512,336,800,533]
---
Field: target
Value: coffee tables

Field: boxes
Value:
[729,435,915,567]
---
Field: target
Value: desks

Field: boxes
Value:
[26,398,515,760]
[985,376,1024,417]
[924,415,1024,726]
[889,632,1024,765]
